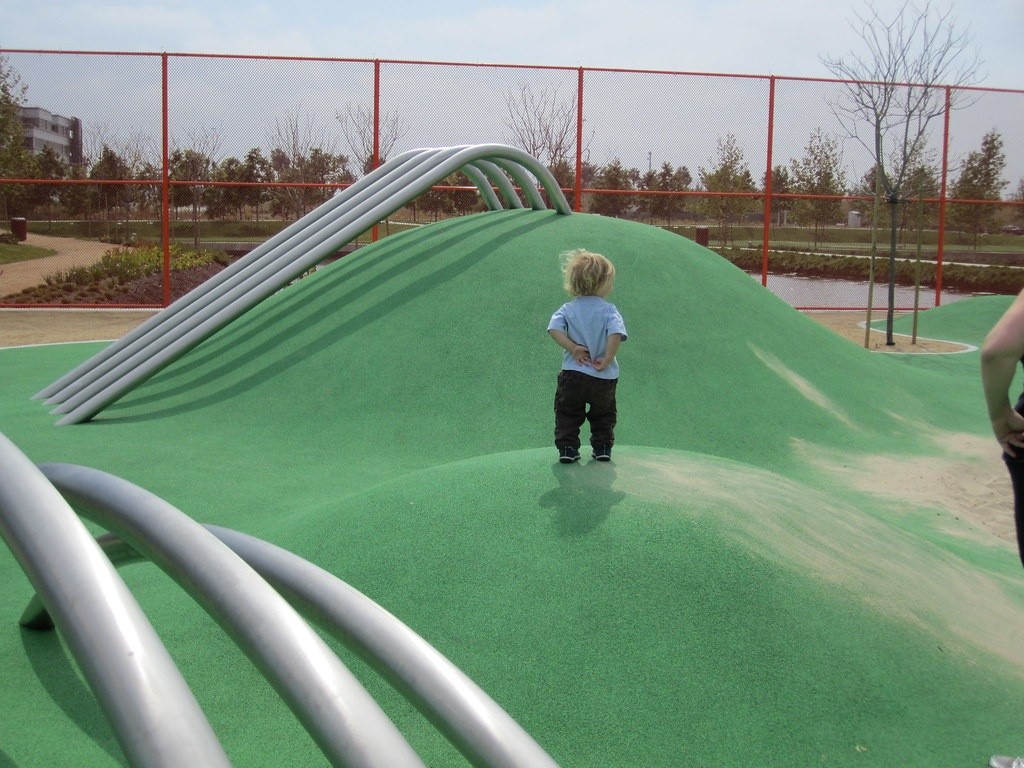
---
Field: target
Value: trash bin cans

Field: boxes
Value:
[10,217,27,241]
[695,226,709,247]
[847,210,862,226]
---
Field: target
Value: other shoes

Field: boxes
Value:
[592,447,612,459]
[559,449,581,462]
[988,754,1024,768]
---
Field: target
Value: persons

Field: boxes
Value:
[546,251,629,463]
[980,286,1024,768]
